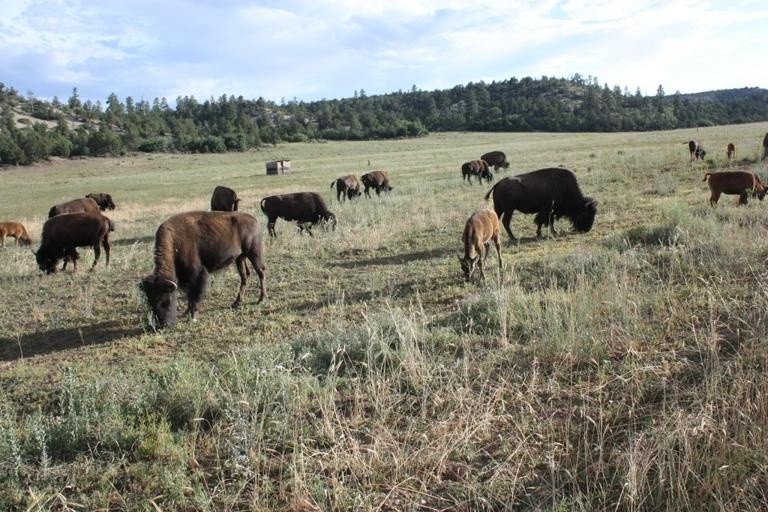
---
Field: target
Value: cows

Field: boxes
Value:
[480,150,510,174]
[330,174,362,202]
[484,167,599,241]
[462,159,494,186]
[137,184,336,333]
[682,133,768,161]
[0,191,116,274]
[702,170,768,208]
[360,170,393,199]
[456,208,503,282]
